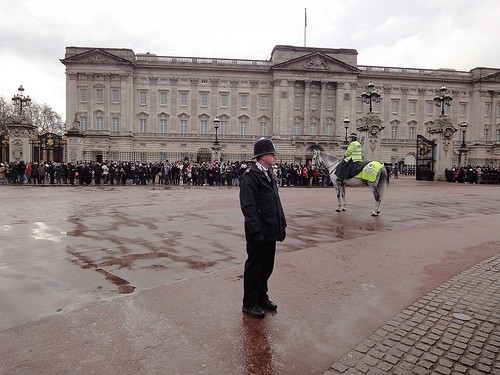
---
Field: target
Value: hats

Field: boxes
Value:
[250,138,276,160]
[348,132,358,138]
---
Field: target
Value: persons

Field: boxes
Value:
[445,161,500,185]
[380,161,416,183]
[271,158,333,187]
[334,133,372,180]
[239,139,287,319]
[0,156,247,187]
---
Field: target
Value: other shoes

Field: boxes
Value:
[473,182,475,184]
[465,182,470,184]
[456,181,458,184]
[336,177,344,182]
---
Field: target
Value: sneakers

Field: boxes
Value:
[256,297,277,310]
[242,304,265,319]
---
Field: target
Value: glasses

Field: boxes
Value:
[269,153,276,157]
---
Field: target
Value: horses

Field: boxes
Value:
[309,149,390,217]
